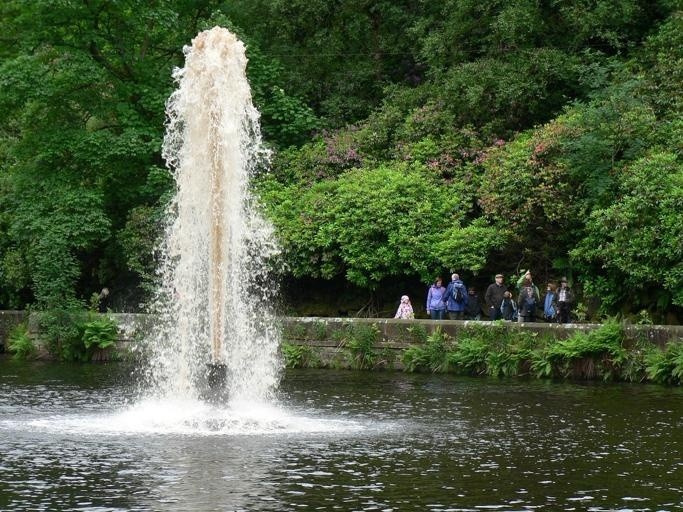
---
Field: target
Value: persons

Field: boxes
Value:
[484,270,576,323]
[441,273,468,320]
[394,295,414,319]
[96,287,112,313]
[426,277,448,320]
[463,286,483,321]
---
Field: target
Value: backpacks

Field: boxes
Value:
[450,283,464,303]
